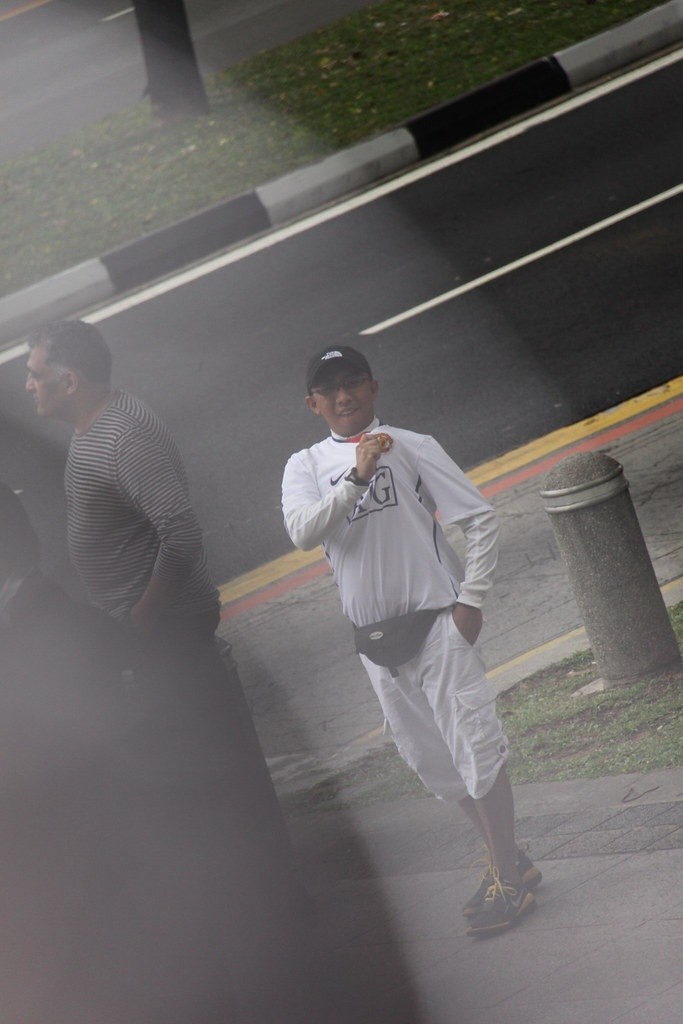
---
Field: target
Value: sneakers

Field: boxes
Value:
[466,865,535,936]
[462,842,542,916]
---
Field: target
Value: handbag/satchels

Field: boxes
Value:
[351,609,443,678]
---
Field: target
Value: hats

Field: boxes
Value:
[307,345,372,389]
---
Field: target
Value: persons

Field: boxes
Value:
[25,315,223,684]
[280,347,540,939]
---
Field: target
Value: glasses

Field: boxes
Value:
[311,373,370,396]
